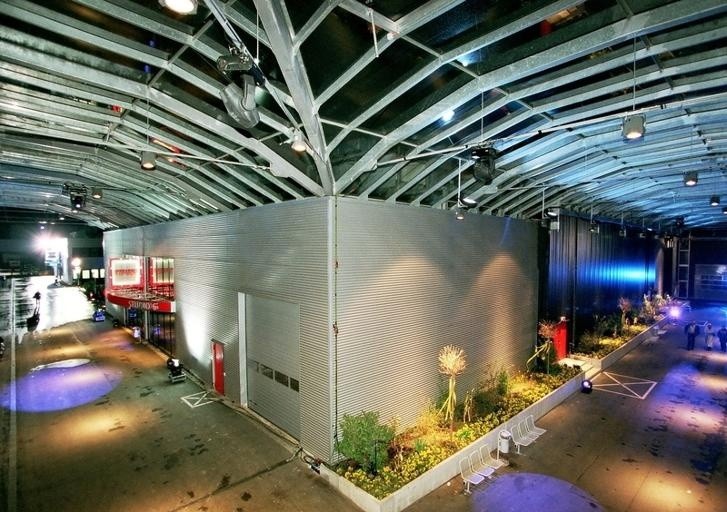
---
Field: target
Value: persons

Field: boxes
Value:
[718,325,726,351]
[703,323,714,350]
[684,320,699,350]
[34,291,41,305]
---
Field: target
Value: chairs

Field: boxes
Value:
[459,415,547,494]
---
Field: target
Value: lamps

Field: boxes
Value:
[140,65,157,169]
[455,159,465,221]
[69,186,85,209]
[291,130,308,152]
[219,72,261,129]
[684,125,727,214]
[91,147,102,199]
[621,35,645,140]
[472,147,498,184]
